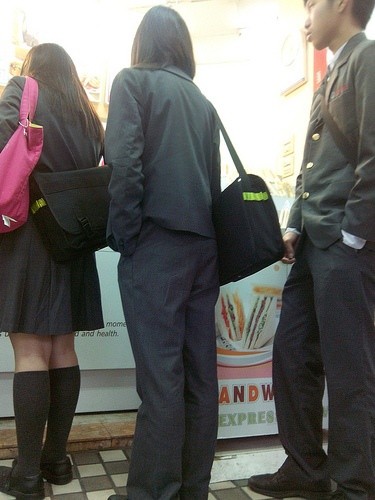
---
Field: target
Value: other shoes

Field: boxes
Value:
[107,494,128,500]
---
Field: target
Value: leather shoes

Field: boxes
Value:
[13,455,73,486]
[248,467,330,500]
[0,466,45,500]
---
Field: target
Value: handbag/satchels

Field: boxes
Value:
[1,76,44,233]
[215,174,286,287]
[28,165,113,253]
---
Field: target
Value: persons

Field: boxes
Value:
[245,0,375,500]
[103,5,220,500]
[0,42,106,500]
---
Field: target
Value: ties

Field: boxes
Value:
[310,65,330,127]
